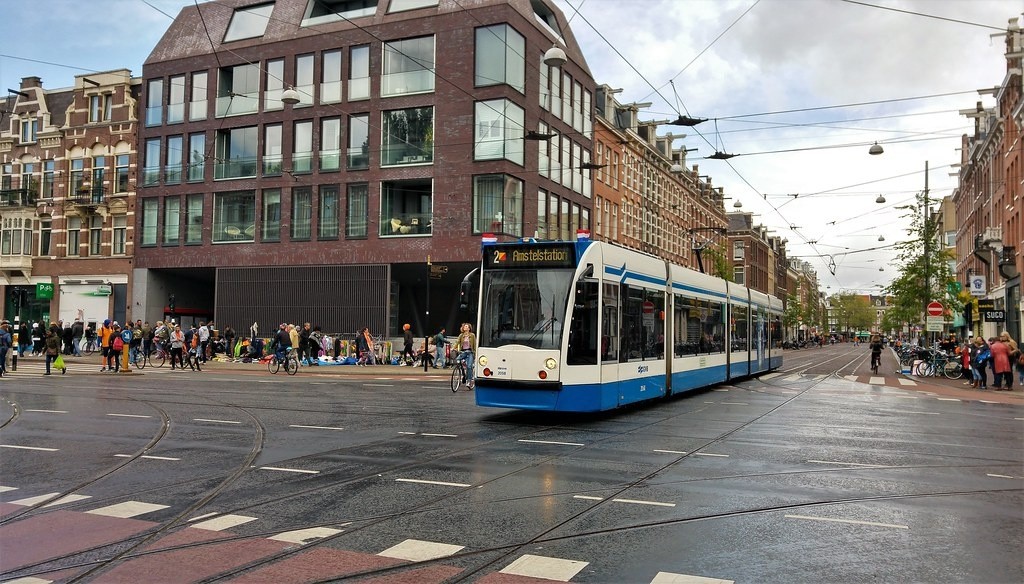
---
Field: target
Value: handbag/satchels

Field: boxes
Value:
[52,354,65,370]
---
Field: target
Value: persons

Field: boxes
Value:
[42,329,66,375]
[937,335,959,354]
[918,338,922,347]
[225,324,234,356]
[85,324,93,352]
[880,337,887,348]
[658,332,664,343]
[418,337,436,367]
[1016,343,1024,385]
[356,327,376,367]
[400,324,418,368]
[532,307,561,331]
[433,327,450,369]
[97,319,216,372]
[0,318,14,377]
[451,323,476,388]
[869,335,884,370]
[700,337,704,353]
[854,337,859,346]
[271,323,324,369]
[814,334,824,348]
[960,332,1018,390]
[18,318,83,357]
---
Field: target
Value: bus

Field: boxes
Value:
[459,227,785,412]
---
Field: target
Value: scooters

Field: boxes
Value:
[782,336,835,350]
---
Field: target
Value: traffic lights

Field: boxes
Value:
[21,290,28,307]
[169,297,174,311]
[12,290,19,307]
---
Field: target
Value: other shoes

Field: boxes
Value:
[171,367,175,370]
[443,366,449,369]
[199,361,203,364]
[100,367,106,372]
[29,353,34,356]
[38,354,43,356]
[44,373,51,375]
[62,367,66,374]
[963,380,974,386]
[309,361,315,367]
[433,367,439,369]
[871,367,873,370]
[1008,387,1013,391]
[994,387,1002,391]
[878,362,881,365]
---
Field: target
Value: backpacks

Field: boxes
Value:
[113,333,123,351]
[431,335,437,345]
[191,334,198,349]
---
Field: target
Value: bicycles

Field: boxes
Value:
[82,329,100,356]
[118,344,146,370]
[874,356,880,374]
[896,343,965,380]
[148,338,190,369]
[450,349,476,392]
[268,347,299,375]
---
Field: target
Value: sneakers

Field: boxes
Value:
[413,362,418,368]
[466,381,471,387]
[400,363,407,367]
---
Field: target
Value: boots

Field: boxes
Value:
[979,380,983,386]
[970,379,978,388]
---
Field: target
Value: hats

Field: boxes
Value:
[33,323,38,327]
[104,319,110,326]
[403,324,410,330]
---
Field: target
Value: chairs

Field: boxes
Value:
[245,225,254,236]
[225,226,240,234]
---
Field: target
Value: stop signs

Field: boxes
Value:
[927,302,944,316]
